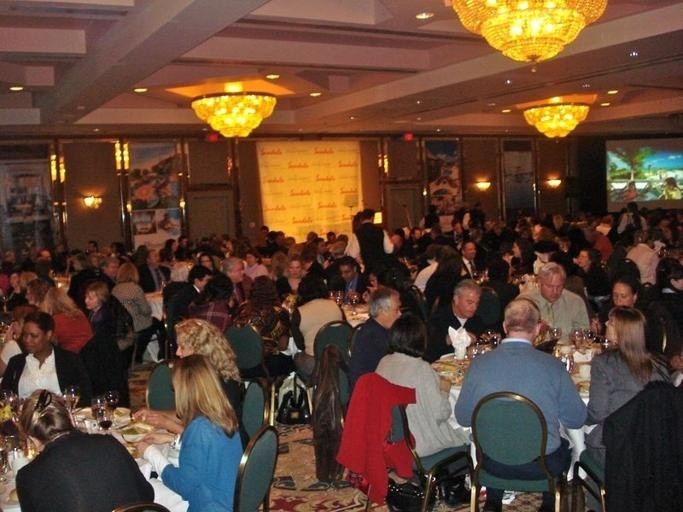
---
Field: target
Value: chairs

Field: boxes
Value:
[234,424,278,510]
[574,386,661,511]
[640,283,654,303]
[314,321,353,405]
[244,381,265,434]
[145,362,175,410]
[361,373,473,511]
[620,259,642,282]
[469,393,564,511]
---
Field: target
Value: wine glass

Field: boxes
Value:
[471,267,535,288]
[0,385,120,486]
[328,289,361,316]
[469,326,612,375]
[159,280,167,295]
[52,272,70,288]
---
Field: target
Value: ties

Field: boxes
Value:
[469,260,477,279]
[545,302,554,325]
[154,268,162,291]
[235,284,243,302]
[320,256,324,264]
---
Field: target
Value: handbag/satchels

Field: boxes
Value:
[386,470,436,511]
[277,389,311,424]
[278,372,311,414]
[92,367,129,408]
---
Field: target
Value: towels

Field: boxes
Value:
[448,328,471,361]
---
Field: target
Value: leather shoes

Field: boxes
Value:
[443,483,471,504]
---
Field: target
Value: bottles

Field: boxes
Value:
[0,321,6,345]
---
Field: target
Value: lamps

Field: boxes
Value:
[84,196,103,209]
[191,91,278,137]
[523,102,589,139]
[453,0,608,63]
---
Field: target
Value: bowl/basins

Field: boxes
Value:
[121,424,152,443]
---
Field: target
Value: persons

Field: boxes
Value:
[658,177,682,200]
[372,312,469,458]
[453,297,588,512]
[136,352,245,511]
[132,318,248,437]
[12,388,156,512]
[0,309,96,410]
[584,304,665,468]
[1,201,682,404]
